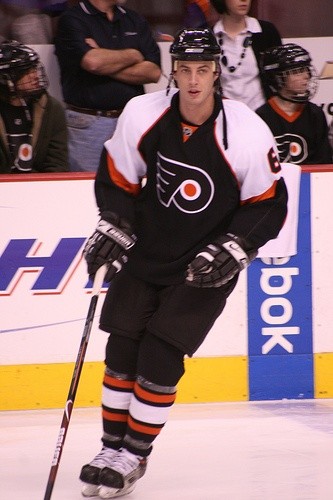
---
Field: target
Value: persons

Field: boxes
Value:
[54,0,162,171]
[211,0,282,111]
[0,0,64,45]
[80,28,288,499]
[0,41,69,173]
[252,43,333,165]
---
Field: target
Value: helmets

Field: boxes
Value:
[169,28,222,61]
[0,39,48,99]
[264,41,312,92]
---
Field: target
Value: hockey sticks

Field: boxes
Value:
[42,260,105,500]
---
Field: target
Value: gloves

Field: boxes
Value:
[85,211,141,282]
[186,231,260,289]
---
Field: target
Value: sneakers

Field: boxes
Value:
[79,445,149,499]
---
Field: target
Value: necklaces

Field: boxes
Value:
[218,31,252,73]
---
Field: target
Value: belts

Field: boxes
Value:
[66,105,121,119]
